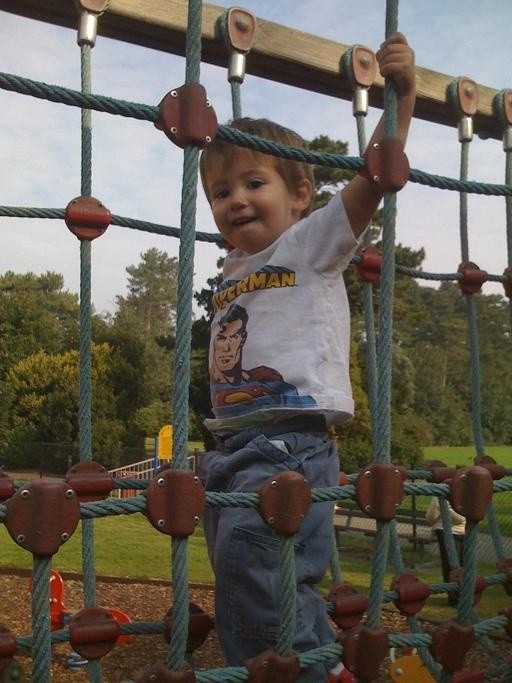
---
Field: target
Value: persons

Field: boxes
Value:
[192,28,420,683]
[209,303,317,417]
